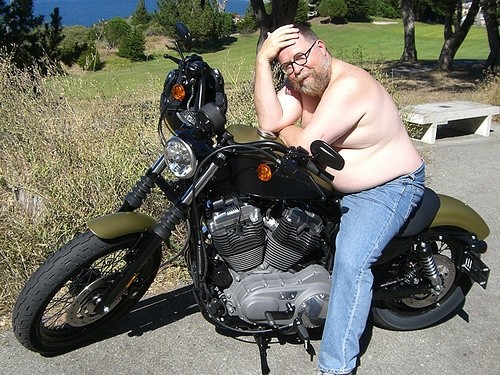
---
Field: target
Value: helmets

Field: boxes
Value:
[158,66,227,142]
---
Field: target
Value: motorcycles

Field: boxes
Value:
[11,21,491,375]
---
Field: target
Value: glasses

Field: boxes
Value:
[278,40,320,75]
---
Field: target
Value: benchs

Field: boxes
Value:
[396,101,500,145]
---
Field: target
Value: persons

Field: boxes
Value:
[254,22,425,375]
